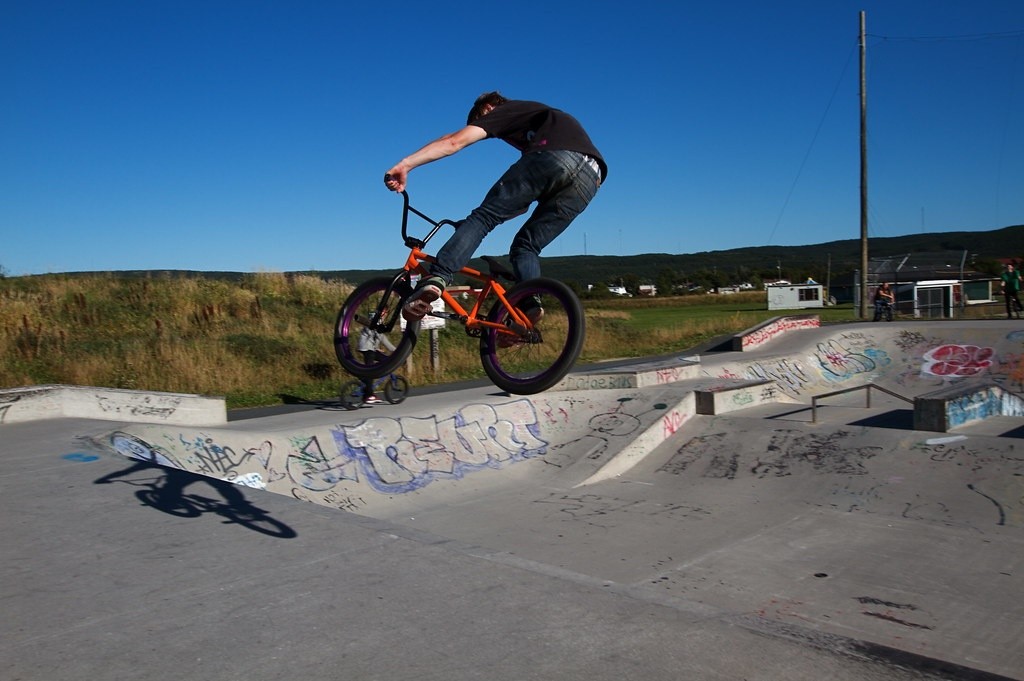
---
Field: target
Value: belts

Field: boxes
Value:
[576,152,601,179]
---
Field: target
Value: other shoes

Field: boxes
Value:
[350,391,359,397]
[363,393,383,403]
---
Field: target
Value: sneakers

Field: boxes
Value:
[496,296,544,348]
[402,277,446,321]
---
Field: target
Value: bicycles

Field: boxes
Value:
[876,296,894,323]
[338,355,409,411]
[334,172,587,398]
[1001,286,1024,319]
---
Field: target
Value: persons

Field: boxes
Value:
[873,280,895,322]
[356,304,397,403]
[1000,264,1024,319]
[384,92,607,347]
